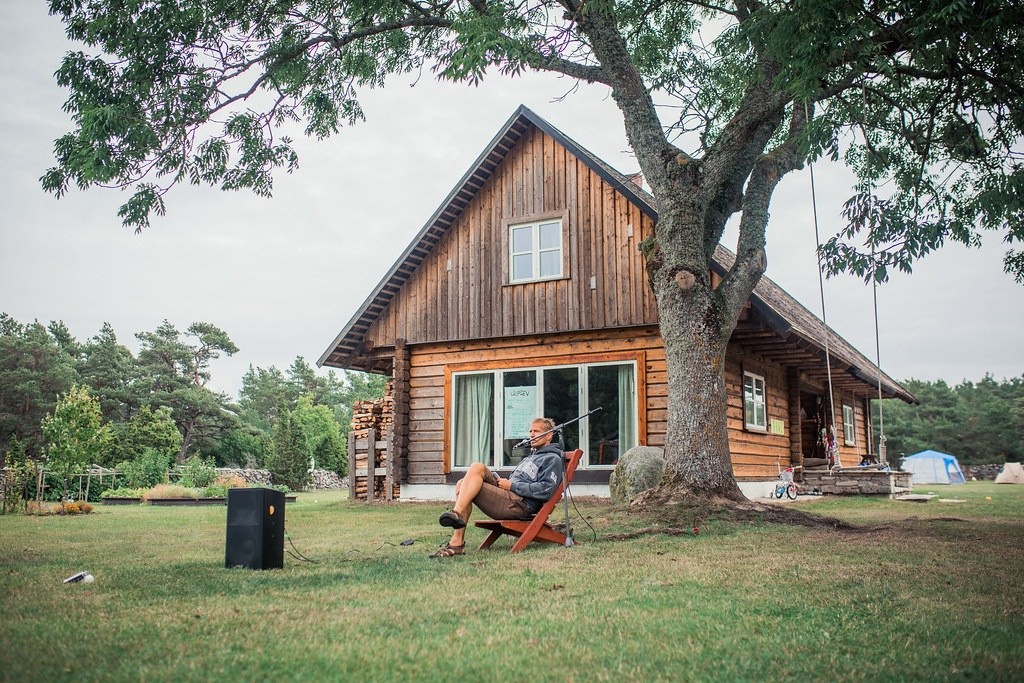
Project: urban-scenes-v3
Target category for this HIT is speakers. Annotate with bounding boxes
[225,488,285,570]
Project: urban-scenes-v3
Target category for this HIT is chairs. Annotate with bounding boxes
[599,440,618,464]
[475,447,585,553]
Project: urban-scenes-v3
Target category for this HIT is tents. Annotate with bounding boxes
[995,462,1024,484]
[899,450,967,484]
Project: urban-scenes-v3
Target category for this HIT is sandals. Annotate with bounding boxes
[429,541,466,558]
[439,510,466,529]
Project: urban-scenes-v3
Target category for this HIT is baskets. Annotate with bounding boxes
[782,472,793,482]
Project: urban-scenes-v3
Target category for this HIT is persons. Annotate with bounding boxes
[897,452,906,480]
[429,417,565,558]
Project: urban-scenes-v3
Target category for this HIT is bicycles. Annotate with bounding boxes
[769,462,802,501]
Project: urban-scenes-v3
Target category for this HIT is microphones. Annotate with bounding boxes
[513,439,528,450]
